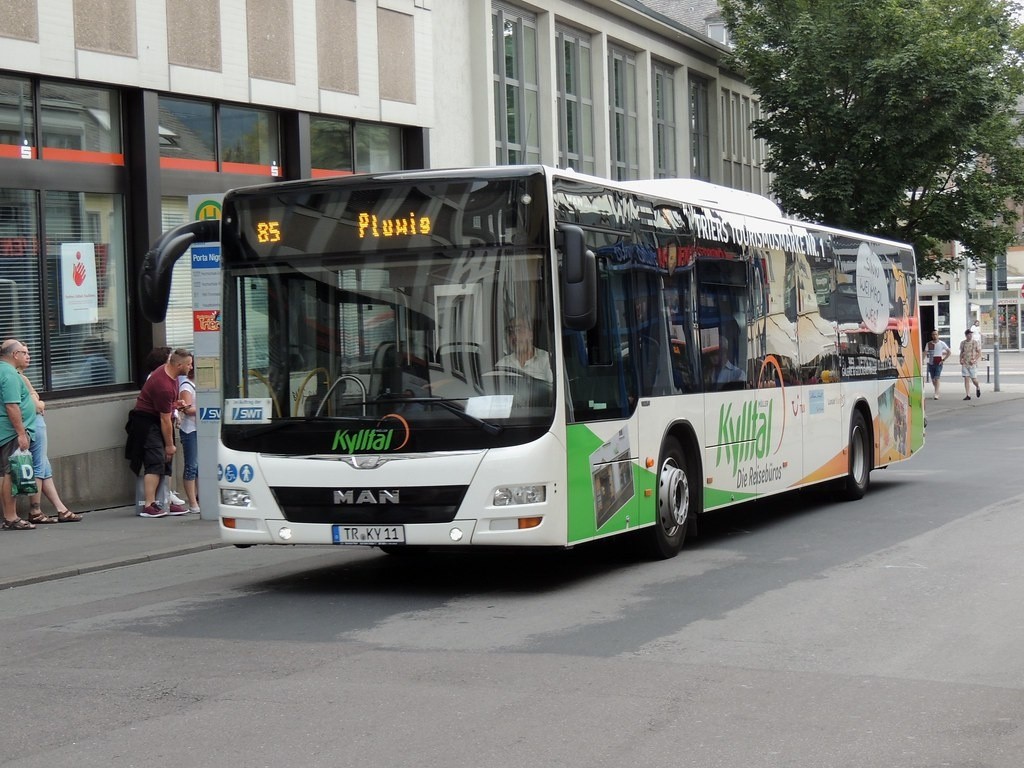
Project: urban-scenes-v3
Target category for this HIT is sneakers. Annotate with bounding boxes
[139,502,167,517]
[169,491,185,505]
[168,505,189,516]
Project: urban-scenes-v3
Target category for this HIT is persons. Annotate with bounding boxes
[921,329,951,400]
[0,339,83,531]
[970,320,983,359]
[124,347,200,517]
[700,334,745,384]
[495,315,553,405]
[959,329,981,400]
[757,367,822,388]
[66,337,114,384]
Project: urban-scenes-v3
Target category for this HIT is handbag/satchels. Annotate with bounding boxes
[8,447,38,497]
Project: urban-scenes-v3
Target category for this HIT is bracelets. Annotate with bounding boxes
[942,359,945,362]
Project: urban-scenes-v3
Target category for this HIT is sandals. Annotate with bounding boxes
[58,509,83,522]
[28,512,58,523]
[3,517,36,530]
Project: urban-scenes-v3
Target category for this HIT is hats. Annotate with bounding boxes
[965,329,973,335]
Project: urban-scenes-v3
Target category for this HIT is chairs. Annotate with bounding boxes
[436,342,484,389]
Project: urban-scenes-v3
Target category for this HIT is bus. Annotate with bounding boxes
[136,162,928,560]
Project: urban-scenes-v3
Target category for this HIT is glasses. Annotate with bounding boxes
[13,351,26,357]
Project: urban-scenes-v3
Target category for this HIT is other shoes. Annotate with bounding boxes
[963,395,971,400]
[189,507,200,514]
[934,395,938,400]
[977,388,980,398]
